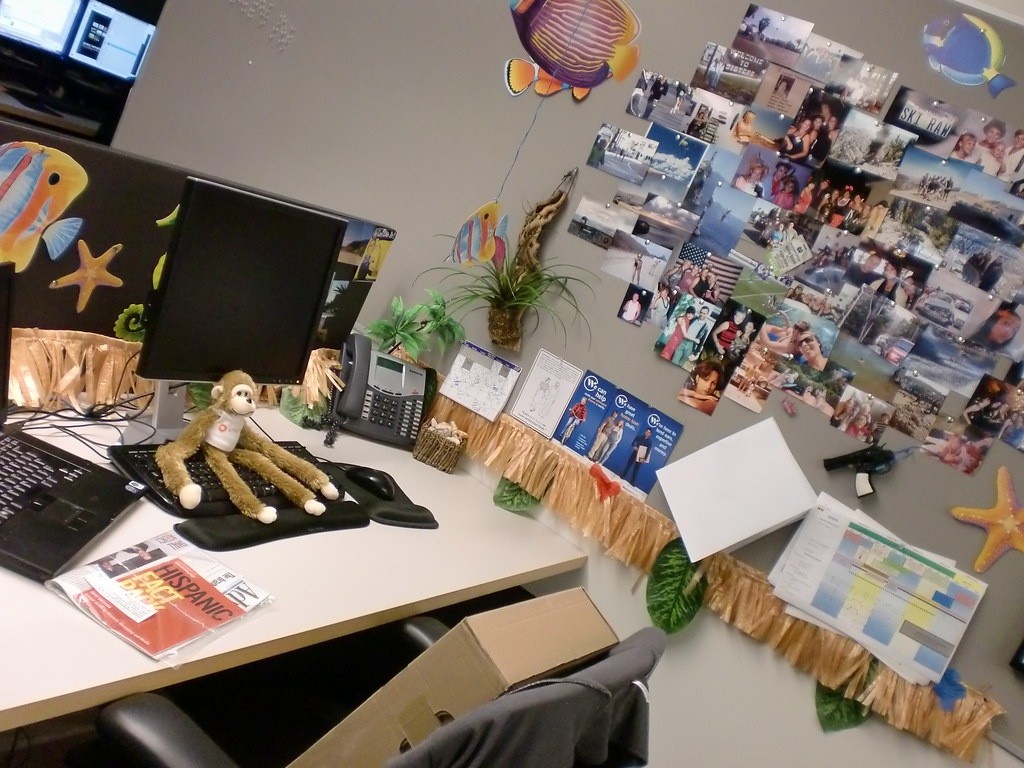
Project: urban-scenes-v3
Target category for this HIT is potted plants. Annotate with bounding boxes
[412,231,603,351]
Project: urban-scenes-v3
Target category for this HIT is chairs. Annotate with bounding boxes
[93,616,668,767]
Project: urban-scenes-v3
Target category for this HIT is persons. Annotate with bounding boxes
[559,46,1024,487]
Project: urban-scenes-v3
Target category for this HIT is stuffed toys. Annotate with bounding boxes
[157,370,340,524]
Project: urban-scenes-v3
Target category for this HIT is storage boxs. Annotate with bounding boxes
[283,586,620,767]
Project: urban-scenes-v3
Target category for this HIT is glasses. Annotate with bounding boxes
[798,336,814,345]
[792,323,805,335]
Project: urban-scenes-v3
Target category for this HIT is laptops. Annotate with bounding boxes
[0,261,151,583]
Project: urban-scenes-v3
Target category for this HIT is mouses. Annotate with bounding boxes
[345,467,396,501]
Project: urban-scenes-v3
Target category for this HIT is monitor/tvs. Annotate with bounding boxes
[0,0,348,443]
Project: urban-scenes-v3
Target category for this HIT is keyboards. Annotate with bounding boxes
[107,440,344,519]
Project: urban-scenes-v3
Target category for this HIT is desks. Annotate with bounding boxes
[0,401,586,734]
